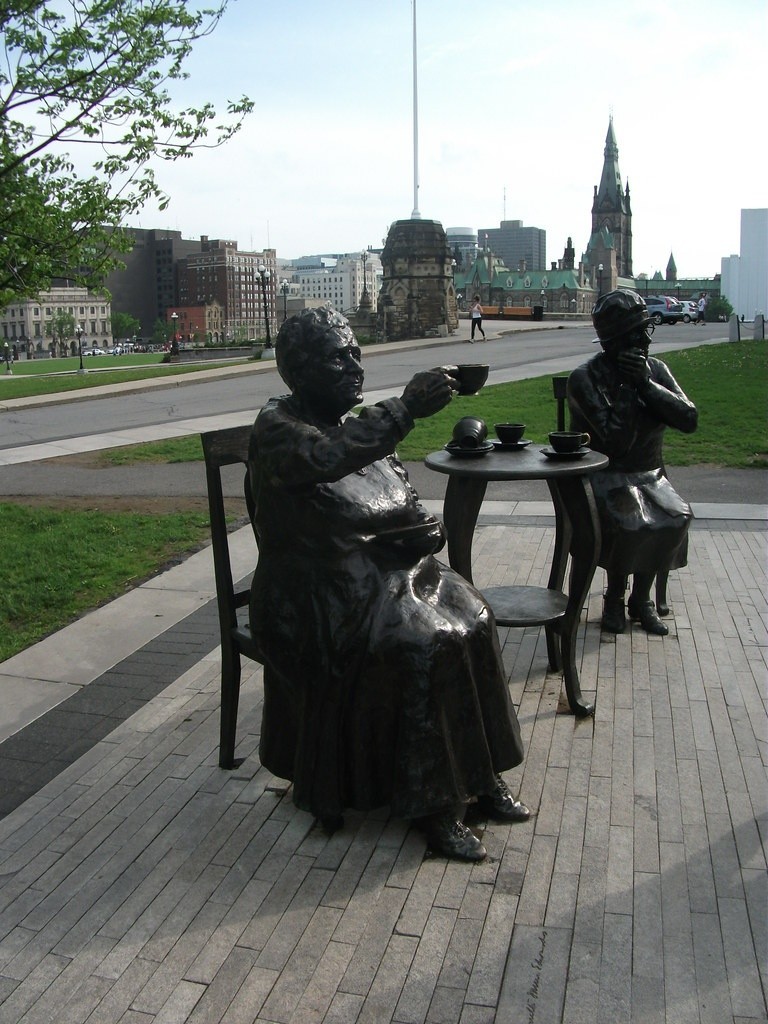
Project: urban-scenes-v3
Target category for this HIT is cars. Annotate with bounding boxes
[679,301,700,324]
[106,347,115,354]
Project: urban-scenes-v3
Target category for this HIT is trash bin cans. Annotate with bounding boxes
[533,305,543,321]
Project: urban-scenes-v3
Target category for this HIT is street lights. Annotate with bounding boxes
[450,259,456,285]
[75,324,84,369]
[457,293,463,309]
[171,312,178,339]
[281,279,290,322]
[674,282,682,300]
[254,265,274,349]
[4,342,10,370]
[360,249,371,297]
[598,264,604,295]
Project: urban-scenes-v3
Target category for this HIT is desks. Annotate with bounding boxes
[423,445,609,716]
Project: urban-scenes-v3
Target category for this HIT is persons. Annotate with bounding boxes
[693,293,707,325]
[468,296,487,344]
[562,289,697,637]
[243,308,536,863]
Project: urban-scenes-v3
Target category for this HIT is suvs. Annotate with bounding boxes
[643,296,684,325]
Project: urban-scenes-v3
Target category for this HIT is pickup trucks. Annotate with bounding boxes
[84,349,105,356]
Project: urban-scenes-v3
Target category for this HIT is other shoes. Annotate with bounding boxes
[468,339,474,344]
[483,334,486,340]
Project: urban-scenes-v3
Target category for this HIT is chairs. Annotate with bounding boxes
[199,426,271,767]
[550,380,671,630]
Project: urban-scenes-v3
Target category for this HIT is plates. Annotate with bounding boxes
[375,520,441,543]
[539,447,592,461]
[444,442,495,459]
[487,439,533,449]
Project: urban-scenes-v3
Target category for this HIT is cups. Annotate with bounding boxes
[447,363,489,397]
[447,416,489,446]
[548,431,590,452]
[494,422,525,443]
